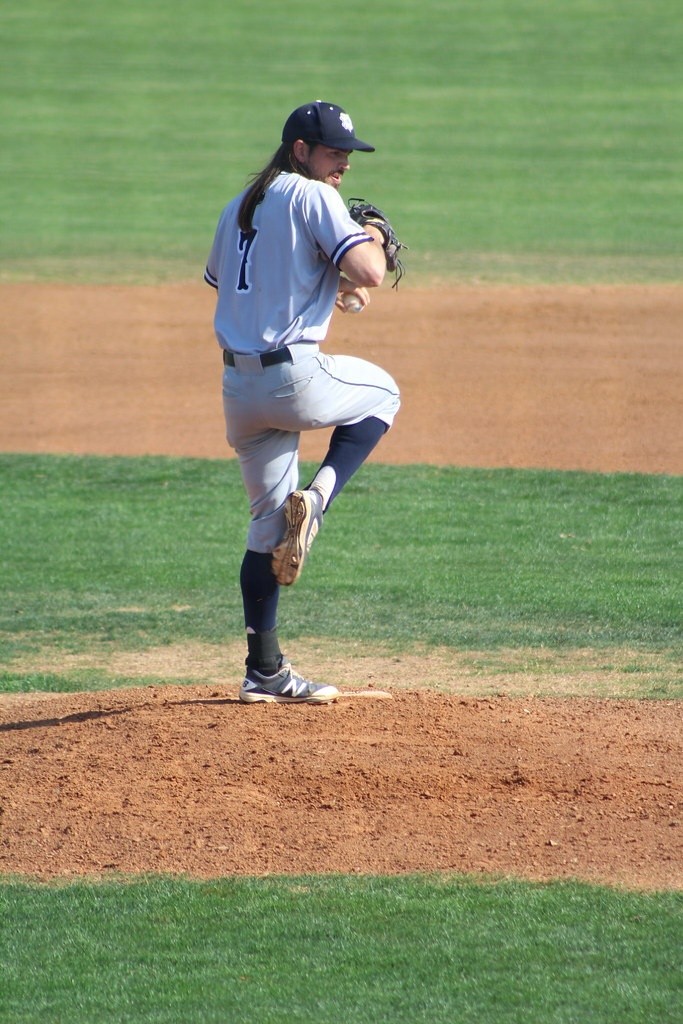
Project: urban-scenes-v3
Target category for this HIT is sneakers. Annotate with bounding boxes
[272,490,323,586]
[239,653,339,705]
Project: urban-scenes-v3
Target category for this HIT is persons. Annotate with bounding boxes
[203,100,408,702]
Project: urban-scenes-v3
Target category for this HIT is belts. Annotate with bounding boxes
[224,346,292,367]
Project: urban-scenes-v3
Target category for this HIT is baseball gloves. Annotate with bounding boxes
[349,201,400,274]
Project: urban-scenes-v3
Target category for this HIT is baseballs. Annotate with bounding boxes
[342,293,364,313]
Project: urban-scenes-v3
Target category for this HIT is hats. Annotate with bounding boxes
[282,100,375,152]
